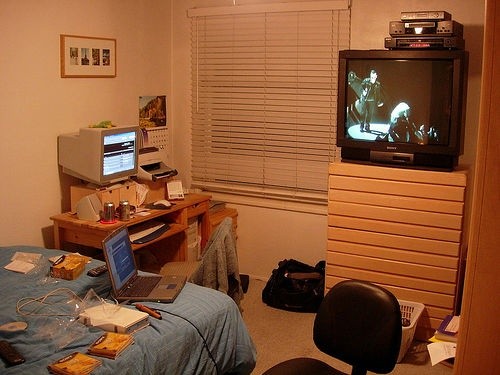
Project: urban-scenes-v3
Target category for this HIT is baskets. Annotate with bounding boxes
[396,298,425,363]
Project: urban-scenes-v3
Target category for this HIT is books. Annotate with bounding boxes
[79,303,150,333]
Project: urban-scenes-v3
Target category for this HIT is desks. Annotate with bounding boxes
[50,192,213,273]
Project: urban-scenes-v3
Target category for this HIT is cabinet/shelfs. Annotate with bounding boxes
[209,206,239,244]
[451,0,500,375]
[322,162,469,330]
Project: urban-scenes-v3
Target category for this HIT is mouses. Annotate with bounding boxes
[152,200,172,209]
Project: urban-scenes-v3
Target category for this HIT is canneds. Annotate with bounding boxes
[103,201,115,222]
[119,200,130,221]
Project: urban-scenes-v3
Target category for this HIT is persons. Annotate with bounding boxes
[391,101,418,131]
[360,70,382,131]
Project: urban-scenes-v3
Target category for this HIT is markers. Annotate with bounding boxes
[136,304,162,320]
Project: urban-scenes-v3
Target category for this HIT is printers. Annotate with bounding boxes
[134,146,179,182]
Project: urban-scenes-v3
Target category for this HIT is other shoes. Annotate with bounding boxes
[365,127,371,133]
[360,128,363,132]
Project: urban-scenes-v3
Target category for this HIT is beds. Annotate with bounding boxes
[0,245,257,375]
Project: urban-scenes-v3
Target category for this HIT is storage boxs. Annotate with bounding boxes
[119,180,139,207]
[397,298,424,366]
[70,184,120,215]
[51,254,87,281]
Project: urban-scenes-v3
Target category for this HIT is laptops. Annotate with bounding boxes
[101,224,187,303]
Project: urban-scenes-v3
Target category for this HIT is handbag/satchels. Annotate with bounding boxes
[376,134,388,141]
[263,259,325,314]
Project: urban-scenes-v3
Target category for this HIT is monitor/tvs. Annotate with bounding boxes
[335,49,469,171]
[58,123,140,190]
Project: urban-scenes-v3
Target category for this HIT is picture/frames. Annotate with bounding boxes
[59,34,118,79]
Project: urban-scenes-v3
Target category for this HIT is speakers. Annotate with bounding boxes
[119,184,137,209]
[76,191,105,222]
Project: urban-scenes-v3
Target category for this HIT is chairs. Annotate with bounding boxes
[160,216,245,319]
[259,279,403,375]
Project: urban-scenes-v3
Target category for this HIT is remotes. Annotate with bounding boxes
[88,264,108,277]
[0,340,25,365]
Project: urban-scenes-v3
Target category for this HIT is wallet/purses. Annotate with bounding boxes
[0,341,26,366]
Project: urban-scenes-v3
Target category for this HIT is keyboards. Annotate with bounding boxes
[126,219,170,244]
